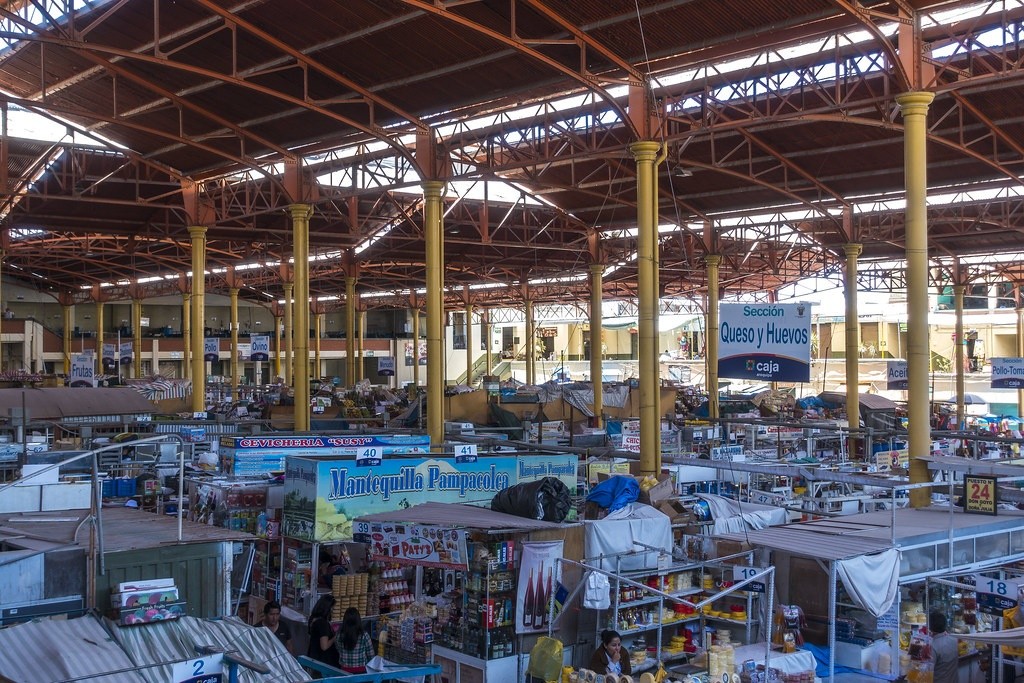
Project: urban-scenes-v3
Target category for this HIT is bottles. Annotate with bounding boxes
[440,617,512,659]
[523,560,553,629]
[359,554,416,612]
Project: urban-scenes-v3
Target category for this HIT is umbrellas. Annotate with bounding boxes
[951,394,987,429]
[972,414,1024,431]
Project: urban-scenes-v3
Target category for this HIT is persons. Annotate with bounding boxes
[590,629,632,679]
[254,395,264,405]
[584,338,590,360]
[917,613,959,683]
[445,385,451,396]
[417,388,426,401]
[207,503,216,525]
[859,447,864,457]
[335,607,374,675]
[1000,430,1022,458]
[938,414,955,440]
[980,432,998,458]
[663,350,673,358]
[306,594,341,679]
[688,350,692,359]
[252,601,292,654]
[482,339,488,349]
[694,353,699,359]
[188,504,202,521]
[307,552,346,589]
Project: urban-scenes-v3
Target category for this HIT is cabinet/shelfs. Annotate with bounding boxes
[594,569,763,674]
[251,533,519,683]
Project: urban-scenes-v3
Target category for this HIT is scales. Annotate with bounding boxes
[669,662,708,683]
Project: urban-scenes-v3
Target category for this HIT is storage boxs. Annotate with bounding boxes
[715,540,753,566]
[111,577,188,627]
[659,500,701,543]
[103,478,136,498]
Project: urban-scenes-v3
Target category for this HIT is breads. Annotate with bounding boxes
[878,600,995,675]
[561,574,816,683]
[330,573,385,622]
